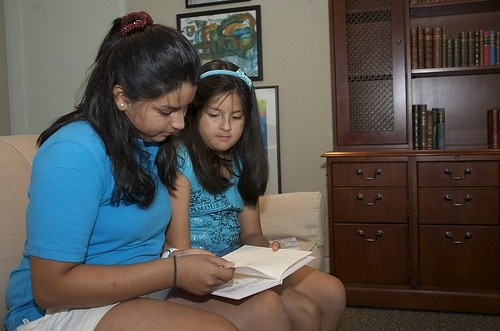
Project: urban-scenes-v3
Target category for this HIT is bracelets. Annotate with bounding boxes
[162,248,177,258]
[173,256,178,289]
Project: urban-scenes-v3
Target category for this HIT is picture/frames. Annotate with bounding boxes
[174,4,264,82]
[253,85,282,195]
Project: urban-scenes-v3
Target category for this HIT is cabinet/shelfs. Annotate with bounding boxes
[329,0,500,148]
[325,157,500,314]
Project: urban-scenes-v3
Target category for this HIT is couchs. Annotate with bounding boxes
[0,134,325,331]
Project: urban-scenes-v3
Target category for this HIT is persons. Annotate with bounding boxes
[4,11,292,331]
[164,59,348,331]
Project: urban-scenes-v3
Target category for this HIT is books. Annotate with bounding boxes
[489,109,500,148]
[410,22,500,69]
[206,242,316,300]
[412,104,445,149]
